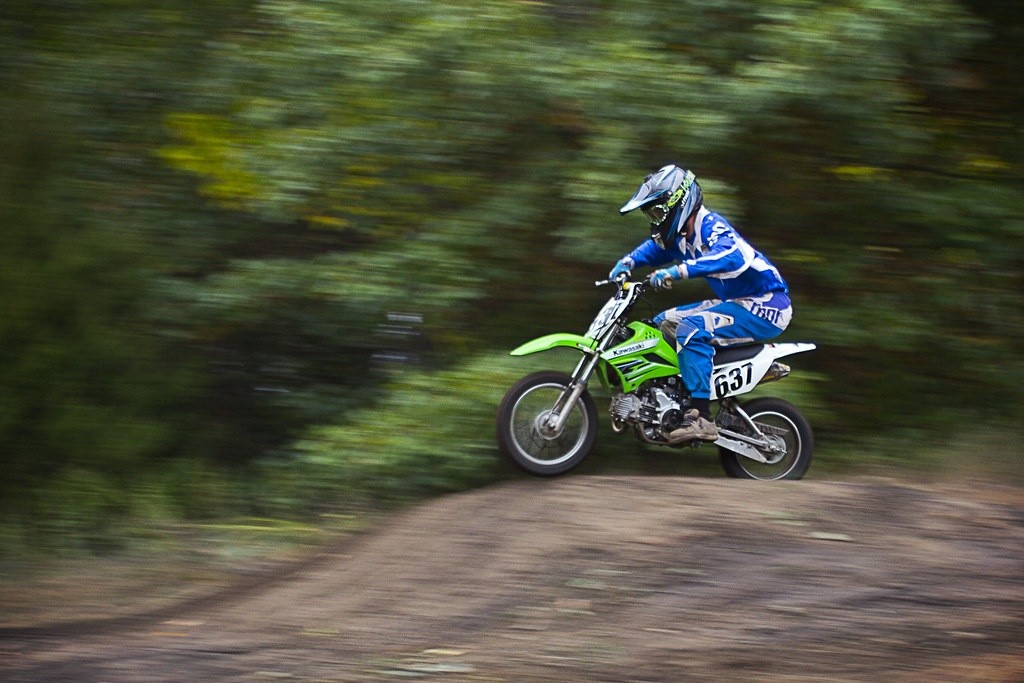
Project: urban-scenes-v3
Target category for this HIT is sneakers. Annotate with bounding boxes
[669,408,719,444]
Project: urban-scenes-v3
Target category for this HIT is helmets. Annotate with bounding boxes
[619,163,702,251]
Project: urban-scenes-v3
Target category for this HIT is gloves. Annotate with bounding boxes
[608,257,635,284]
[647,264,682,294]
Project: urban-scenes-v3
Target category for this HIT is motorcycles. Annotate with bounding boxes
[491,275,815,485]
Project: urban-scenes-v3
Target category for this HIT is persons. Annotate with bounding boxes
[610,165,792,441]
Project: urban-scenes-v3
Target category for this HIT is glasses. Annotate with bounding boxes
[641,205,669,226]
